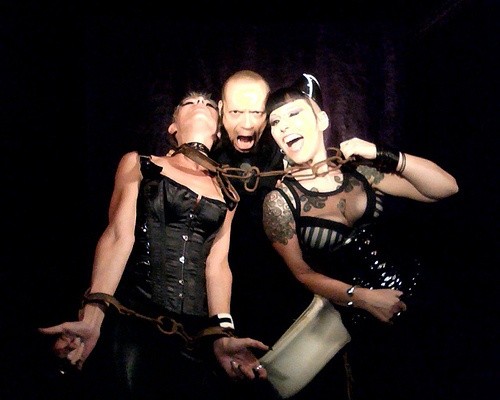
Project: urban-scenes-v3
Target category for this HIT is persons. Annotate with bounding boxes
[36,71,459,383]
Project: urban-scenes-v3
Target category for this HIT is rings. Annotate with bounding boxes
[255,365,262,371]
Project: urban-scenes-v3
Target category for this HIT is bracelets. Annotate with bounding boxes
[78,293,116,320]
[398,152,406,174]
[200,327,233,338]
[204,313,235,329]
[370,142,399,171]
[347,284,360,307]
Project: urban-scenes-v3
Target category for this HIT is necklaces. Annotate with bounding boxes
[172,141,209,157]
